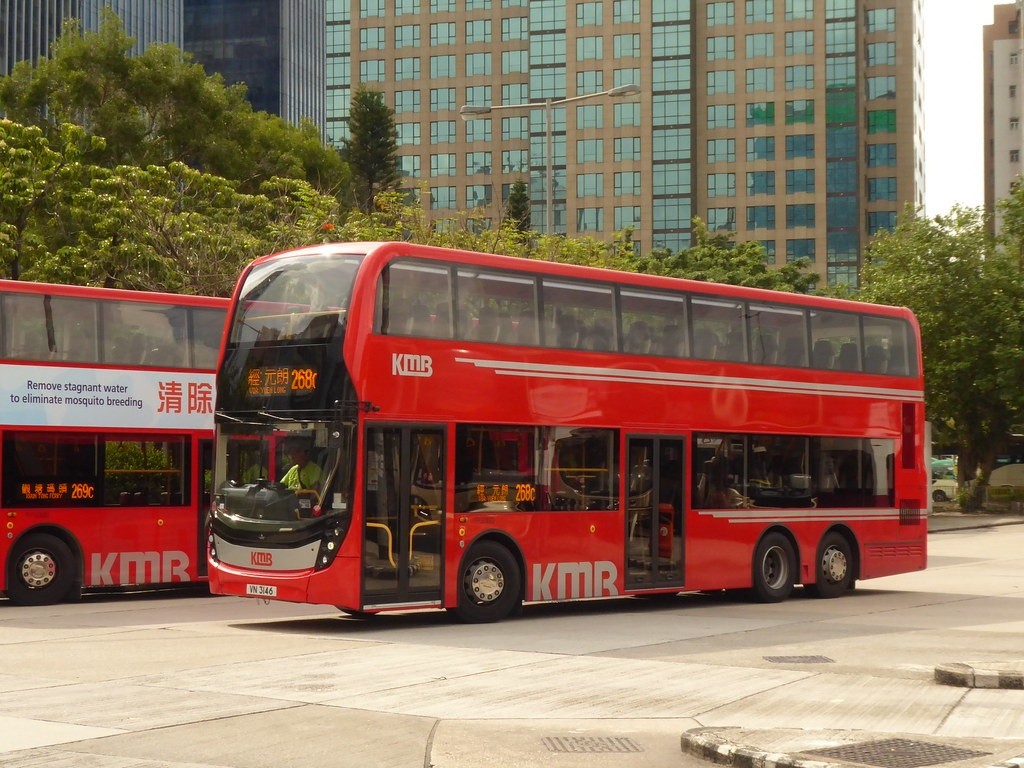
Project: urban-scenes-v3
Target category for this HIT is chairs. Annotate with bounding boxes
[383,296,912,377]
[21,330,184,369]
[121,490,172,507]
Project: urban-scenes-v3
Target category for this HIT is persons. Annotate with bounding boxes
[280,445,324,516]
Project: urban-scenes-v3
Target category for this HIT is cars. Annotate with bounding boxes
[930,455,958,502]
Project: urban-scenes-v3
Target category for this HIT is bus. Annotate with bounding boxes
[0,277,527,606]
[204,239,929,626]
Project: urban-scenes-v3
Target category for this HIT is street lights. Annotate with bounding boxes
[458,83,644,259]
